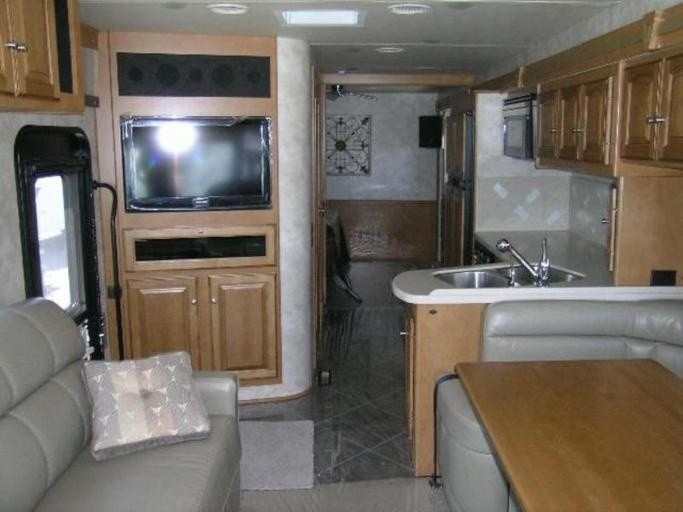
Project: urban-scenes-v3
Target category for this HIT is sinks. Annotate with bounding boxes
[436,272,511,289]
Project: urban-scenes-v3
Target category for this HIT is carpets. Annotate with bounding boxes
[237,419,317,493]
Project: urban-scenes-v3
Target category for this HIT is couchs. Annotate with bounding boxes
[434,300,682,512]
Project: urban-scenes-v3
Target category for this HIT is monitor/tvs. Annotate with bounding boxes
[120,114,273,212]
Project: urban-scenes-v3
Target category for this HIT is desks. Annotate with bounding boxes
[453,357,682,512]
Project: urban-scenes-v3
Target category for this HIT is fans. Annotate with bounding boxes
[324,85,378,102]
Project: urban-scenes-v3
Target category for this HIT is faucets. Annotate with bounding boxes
[496,238,550,287]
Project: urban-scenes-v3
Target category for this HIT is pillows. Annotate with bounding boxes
[81,350,215,462]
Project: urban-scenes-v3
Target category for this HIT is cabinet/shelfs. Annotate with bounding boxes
[0,0,61,112]
[400,301,488,478]
[619,3,683,170]
[537,28,623,178]
[119,266,282,388]
[118,223,277,274]
[600,176,682,286]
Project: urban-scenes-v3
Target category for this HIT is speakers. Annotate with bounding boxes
[418,115,442,149]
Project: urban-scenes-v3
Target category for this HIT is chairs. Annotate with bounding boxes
[0,296,244,512]
